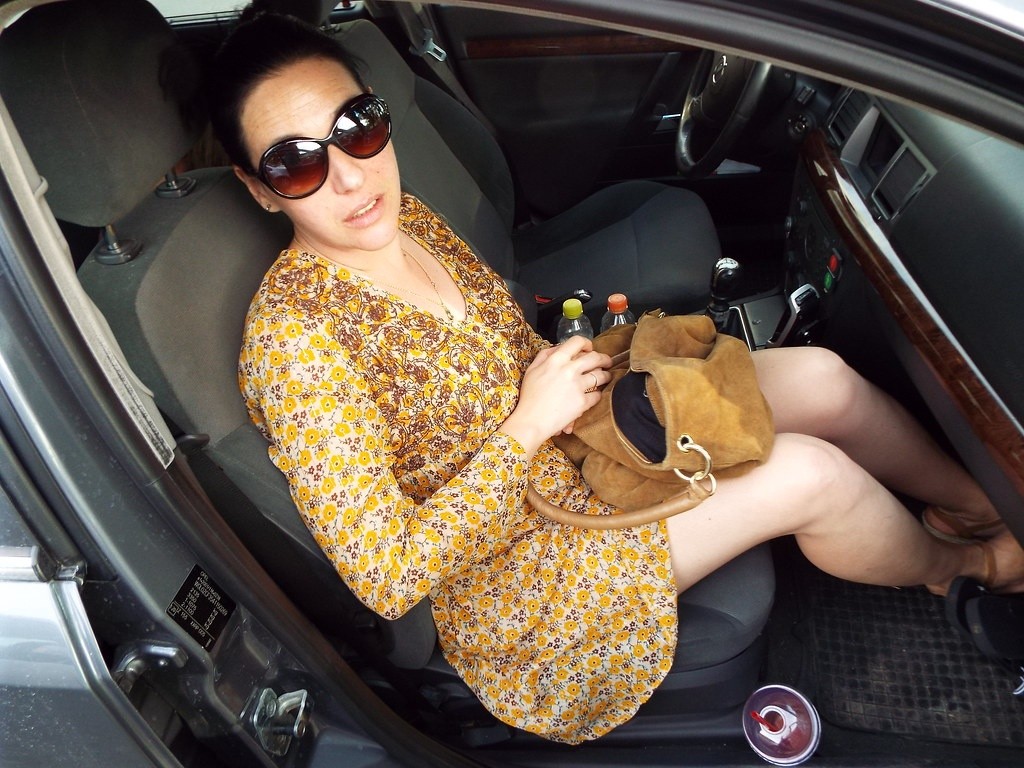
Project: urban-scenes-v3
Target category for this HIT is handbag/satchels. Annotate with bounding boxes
[521,312,775,525]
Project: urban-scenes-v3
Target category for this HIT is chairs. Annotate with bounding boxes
[250,0,720,339]
[2,0,776,677]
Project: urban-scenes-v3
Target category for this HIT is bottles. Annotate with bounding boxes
[601,293,636,334]
[557,298,594,344]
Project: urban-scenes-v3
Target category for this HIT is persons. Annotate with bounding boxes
[157,10,1024,746]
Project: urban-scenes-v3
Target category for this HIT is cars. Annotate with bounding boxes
[0,1,1024,767]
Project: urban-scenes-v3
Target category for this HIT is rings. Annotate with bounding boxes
[585,372,597,392]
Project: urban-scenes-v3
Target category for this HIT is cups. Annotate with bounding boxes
[741,684,822,767]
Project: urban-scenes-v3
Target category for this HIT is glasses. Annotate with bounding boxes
[248,92,397,201]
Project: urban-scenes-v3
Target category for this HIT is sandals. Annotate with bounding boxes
[923,538,1024,597]
[923,503,1007,542]
